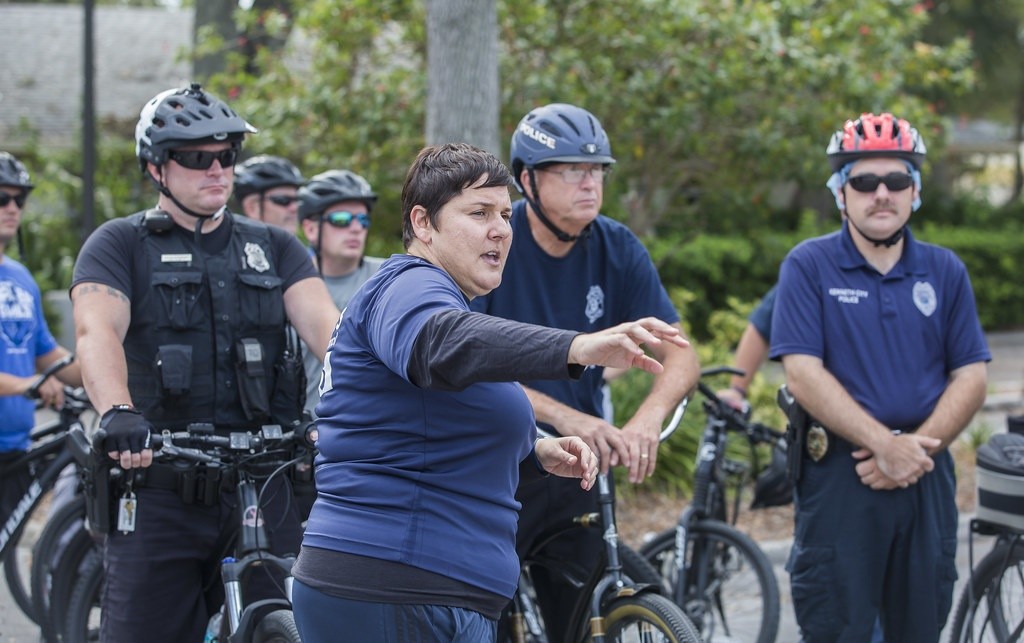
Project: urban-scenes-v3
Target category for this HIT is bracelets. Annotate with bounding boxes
[729,387,746,398]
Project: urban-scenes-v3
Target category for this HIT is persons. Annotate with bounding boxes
[0,152,82,527]
[473,104,699,643]
[295,170,390,419]
[714,284,784,409]
[72,84,342,643]
[769,111,992,643]
[292,145,691,643]
[233,153,318,272]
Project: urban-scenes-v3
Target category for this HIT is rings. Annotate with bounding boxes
[640,454,649,458]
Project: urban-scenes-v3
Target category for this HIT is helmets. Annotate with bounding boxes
[0,151,34,192]
[134,83,259,173]
[510,103,617,183]
[826,112,927,173]
[234,156,307,207]
[297,169,379,225]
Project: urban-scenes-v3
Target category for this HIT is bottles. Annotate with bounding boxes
[202,603,227,643]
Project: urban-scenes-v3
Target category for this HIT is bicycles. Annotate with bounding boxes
[0,355,317,643]
[636,366,795,643]
[498,395,701,643]
[947,415,1024,643]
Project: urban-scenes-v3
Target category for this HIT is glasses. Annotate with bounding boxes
[269,195,297,206]
[848,171,914,193]
[168,148,235,170]
[323,210,372,227]
[0,192,25,208]
[544,164,613,183]
